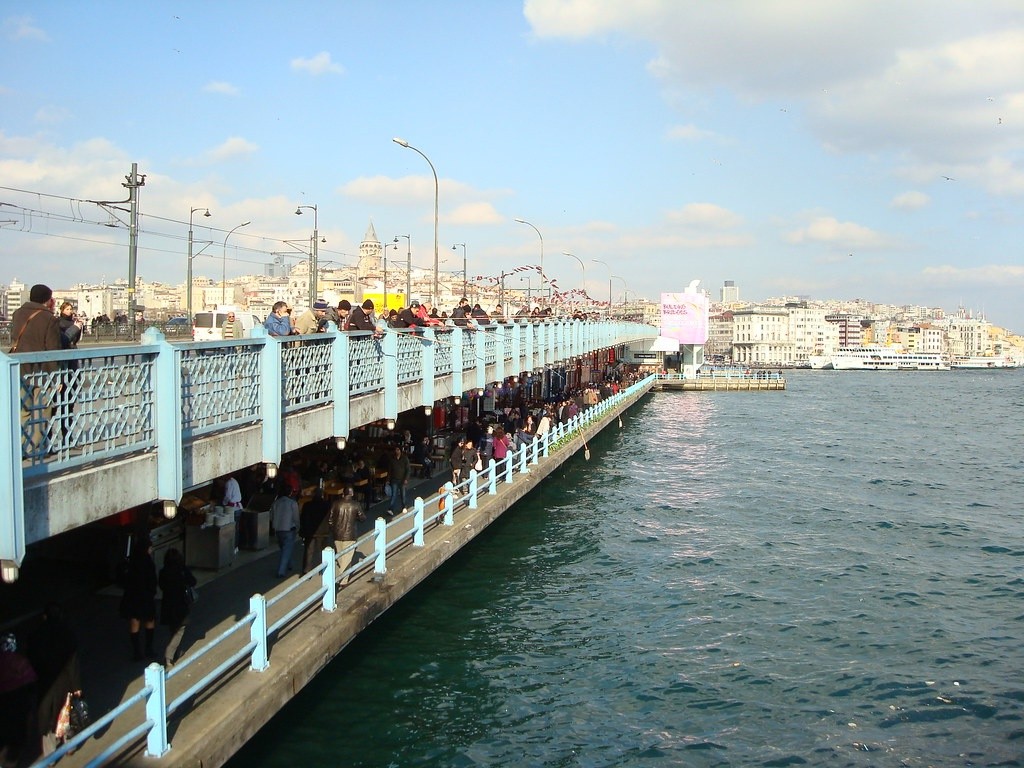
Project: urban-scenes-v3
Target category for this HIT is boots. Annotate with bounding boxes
[131,628,154,662]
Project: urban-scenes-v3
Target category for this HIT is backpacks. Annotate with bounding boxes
[481,441,493,458]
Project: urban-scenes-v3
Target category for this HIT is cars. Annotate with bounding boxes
[164,317,188,333]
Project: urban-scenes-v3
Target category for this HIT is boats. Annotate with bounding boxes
[808,343,1017,371]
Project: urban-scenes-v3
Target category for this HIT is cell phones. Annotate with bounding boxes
[277,310,282,316]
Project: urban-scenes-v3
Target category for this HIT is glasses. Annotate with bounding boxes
[228,316,235,318]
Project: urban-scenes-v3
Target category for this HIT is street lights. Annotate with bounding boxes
[384,242,398,317]
[452,243,467,299]
[514,217,544,310]
[593,259,612,319]
[610,275,637,315]
[561,252,586,290]
[393,234,411,307]
[188,206,212,330]
[308,235,327,311]
[392,137,438,308]
[520,276,530,311]
[294,204,318,304]
[222,221,251,304]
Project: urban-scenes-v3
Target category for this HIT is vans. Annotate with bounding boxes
[191,312,262,357]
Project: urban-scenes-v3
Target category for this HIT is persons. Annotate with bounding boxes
[158,548,198,667]
[222,312,243,376]
[743,369,783,379]
[298,487,333,579]
[119,546,157,661]
[663,369,667,379]
[710,368,714,379]
[264,297,658,515]
[269,484,300,580]
[0,601,77,768]
[206,474,243,555]
[328,487,367,586]
[10,285,85,457]
[696,368,700,379]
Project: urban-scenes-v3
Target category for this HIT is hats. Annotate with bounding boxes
[313,299,328,311]
[362,299,374,310]
[339,300,351,311]
[389,309,398,318]
[30,285,52,303]
[410,301,421,308]
[463,305,471,312]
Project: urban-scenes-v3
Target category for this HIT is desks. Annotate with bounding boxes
[300,471,388,503]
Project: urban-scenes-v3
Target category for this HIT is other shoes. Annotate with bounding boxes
[162,656,173,667]
[63,441,83,450]
[402,508,407,513]
[387,510,393,516]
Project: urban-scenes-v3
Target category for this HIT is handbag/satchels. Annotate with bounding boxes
[385,483,393,497]
[55,691,90,740]
[9,346,16,353]
[184,587,198,605]
[475,453,482,471]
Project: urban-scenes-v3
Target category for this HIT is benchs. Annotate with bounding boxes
[409,461,423,477]
[431,456,444,471]
